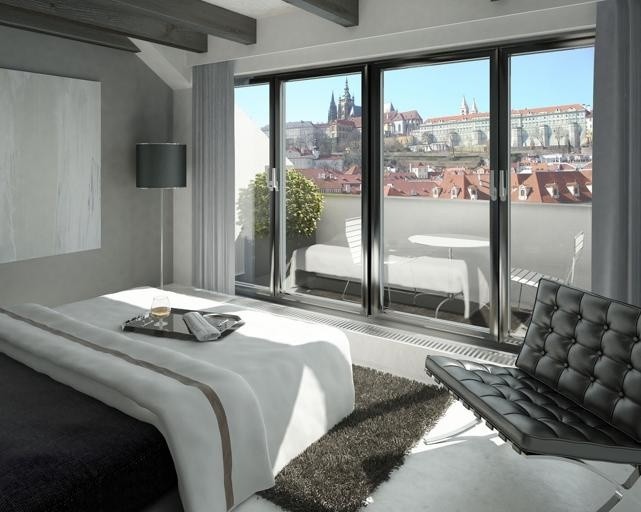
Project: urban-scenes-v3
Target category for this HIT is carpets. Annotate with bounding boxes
[254,361,457,512]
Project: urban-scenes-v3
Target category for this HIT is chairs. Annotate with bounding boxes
[508,230,587,316]
[340,217,396,306]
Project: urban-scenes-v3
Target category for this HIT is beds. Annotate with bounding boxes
[1,283,357,512]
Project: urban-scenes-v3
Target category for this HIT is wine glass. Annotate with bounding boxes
[151,296,171,327]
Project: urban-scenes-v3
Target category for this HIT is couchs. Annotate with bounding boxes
[424,275,641,511]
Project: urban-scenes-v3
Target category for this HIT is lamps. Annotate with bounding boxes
[135,141,188,282]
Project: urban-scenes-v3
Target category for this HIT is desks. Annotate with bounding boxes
[409,233,491,318]
